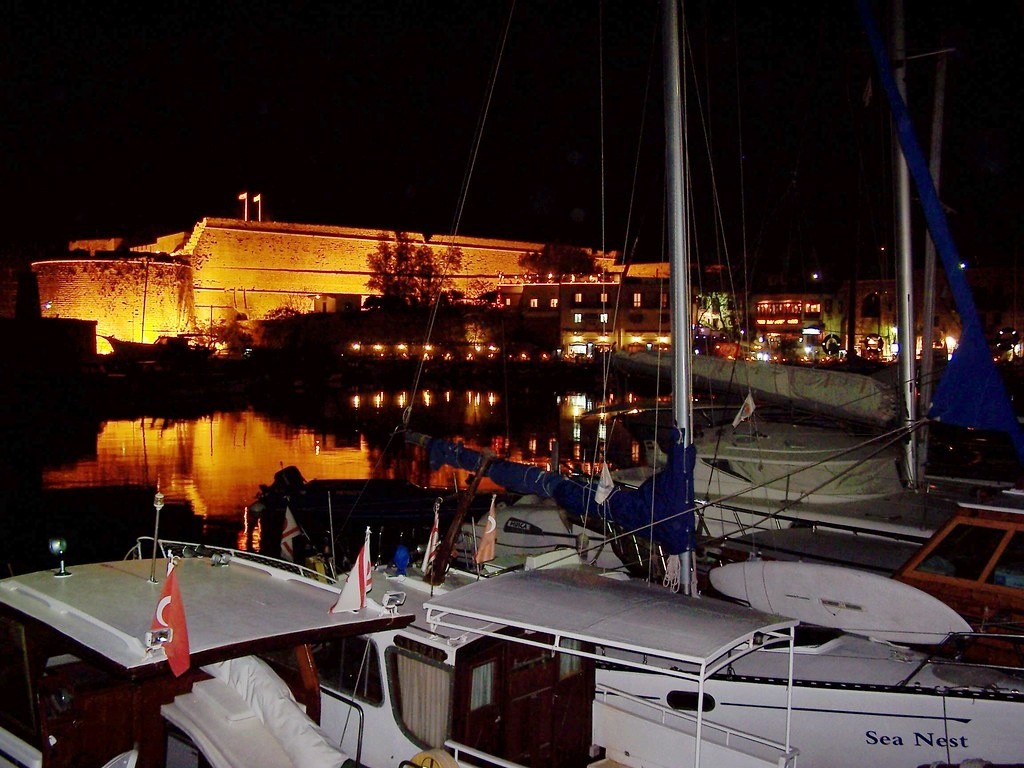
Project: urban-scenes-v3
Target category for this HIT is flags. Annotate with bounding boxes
[595,461,614,505]
[420,514,442,573]
[149,562,190,678]
[278,508,301,559]
[328,533,376,614]
[473,502,495,563]
[733,394,755,428]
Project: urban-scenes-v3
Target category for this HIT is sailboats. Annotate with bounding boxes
[2,1,1024,765]
